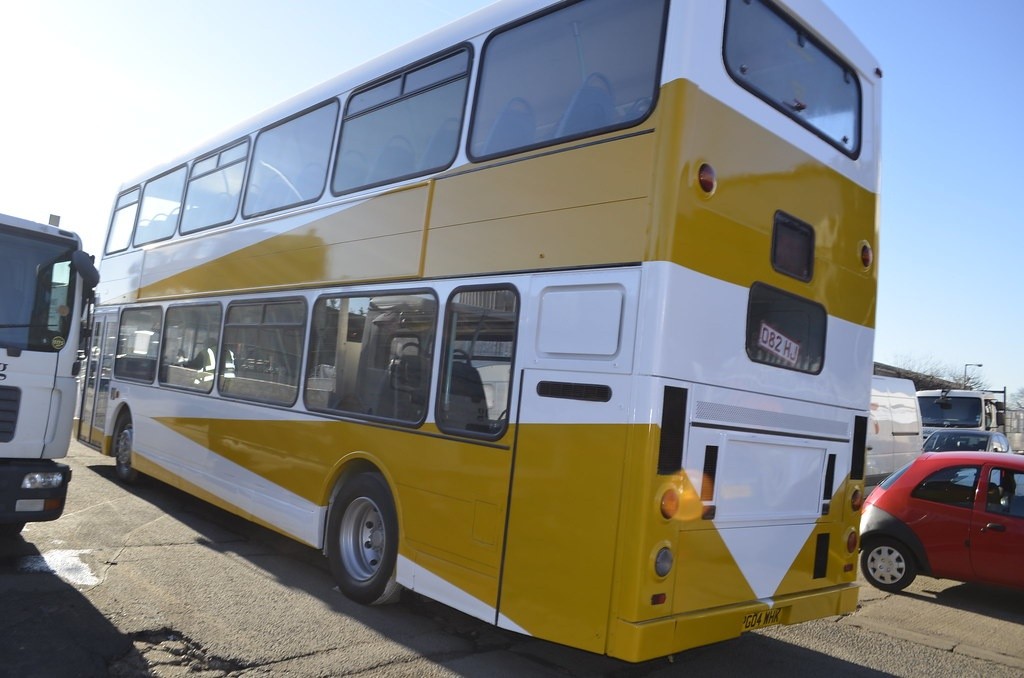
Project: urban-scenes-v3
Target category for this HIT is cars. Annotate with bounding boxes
[922,429,1015,458]
[856,450,1024,602]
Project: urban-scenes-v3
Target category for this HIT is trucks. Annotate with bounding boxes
[859,374,922,510]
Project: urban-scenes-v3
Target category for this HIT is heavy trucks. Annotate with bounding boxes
[0,209,101,537]
[914,386,998,447]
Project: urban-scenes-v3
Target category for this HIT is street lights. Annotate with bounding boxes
[963,363,984,390]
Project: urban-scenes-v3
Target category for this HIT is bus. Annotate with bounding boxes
[79,0,883,662]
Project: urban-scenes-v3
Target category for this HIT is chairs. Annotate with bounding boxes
[129,70,653,250]
[914,478,1024,518]
[371,338,489,434]
